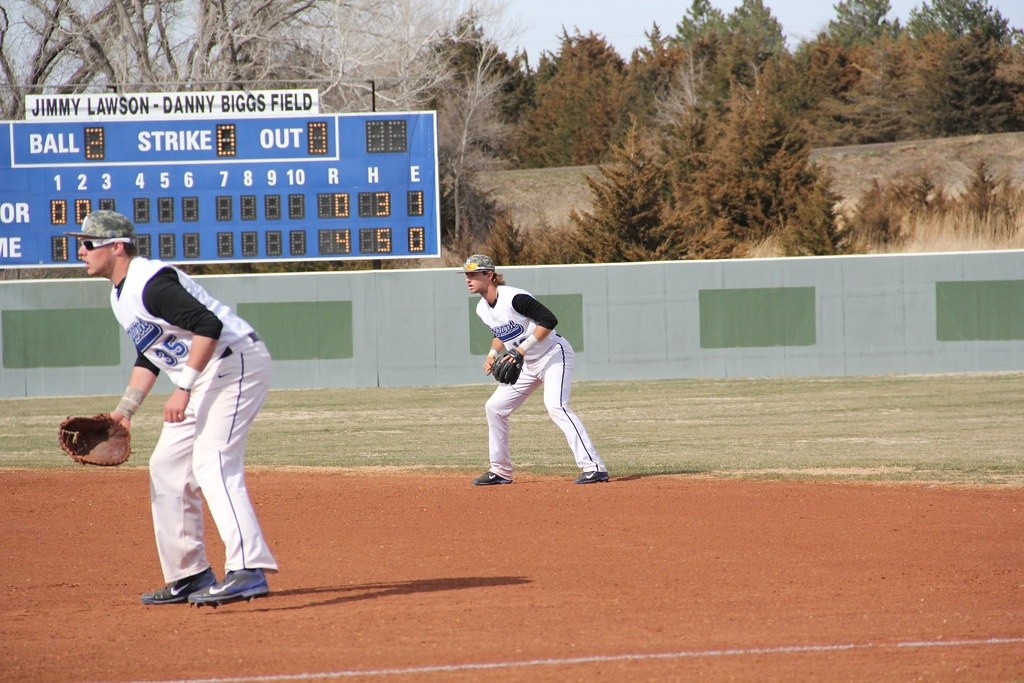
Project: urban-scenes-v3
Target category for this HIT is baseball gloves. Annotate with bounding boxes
[489,349,525,385]
[58,411,134,466]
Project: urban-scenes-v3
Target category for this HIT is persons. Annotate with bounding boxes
[62,210,281,606]
[456,253,609,485]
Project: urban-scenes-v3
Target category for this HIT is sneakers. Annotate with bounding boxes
[189,567,269,605]
[472,472,513,485]
[141,566,217,604]
[574,471,608,484]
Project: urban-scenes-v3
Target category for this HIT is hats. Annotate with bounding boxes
[459,253,495,274]
[65,210,136,244]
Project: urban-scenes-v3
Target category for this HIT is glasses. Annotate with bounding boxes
[80,238,130,251]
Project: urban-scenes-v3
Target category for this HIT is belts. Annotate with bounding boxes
[220,331,261,359]
